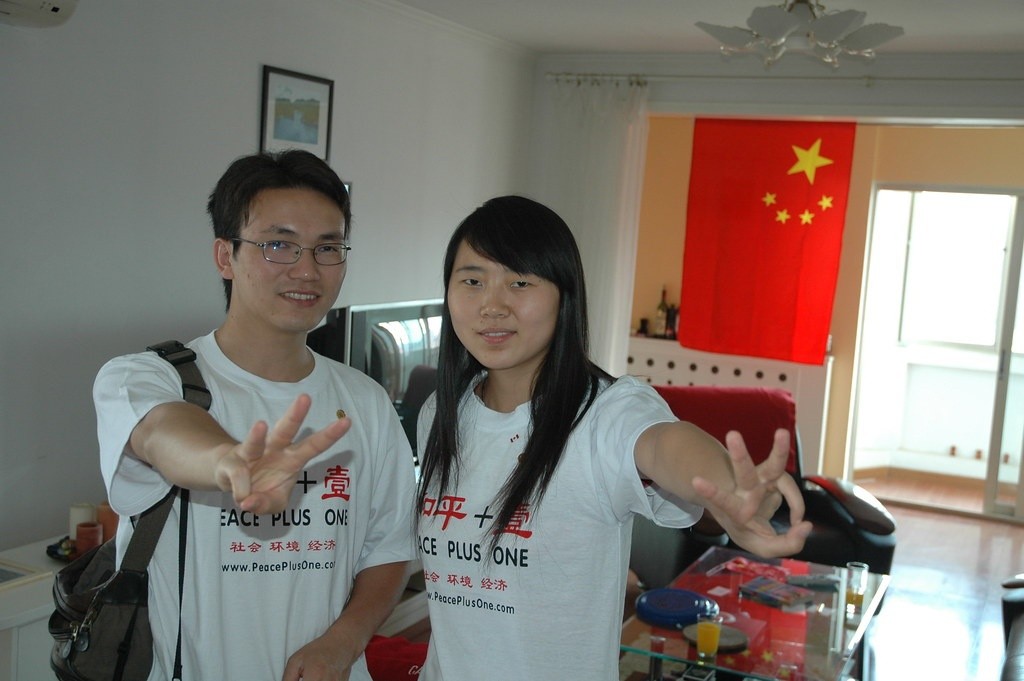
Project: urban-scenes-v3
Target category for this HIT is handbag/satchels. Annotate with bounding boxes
[48,339,211,681]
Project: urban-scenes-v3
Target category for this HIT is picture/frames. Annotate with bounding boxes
[258,65,334,162]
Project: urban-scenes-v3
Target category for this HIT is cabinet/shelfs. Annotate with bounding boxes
[0,533,69,681]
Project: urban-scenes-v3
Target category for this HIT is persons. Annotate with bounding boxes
[93,144,424,681]
[409,194,813,681]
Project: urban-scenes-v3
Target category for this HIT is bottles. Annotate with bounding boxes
[653,285,667,337]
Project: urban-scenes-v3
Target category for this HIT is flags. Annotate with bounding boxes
[676,116,858,366]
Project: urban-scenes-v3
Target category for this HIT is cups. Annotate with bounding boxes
[97,503,119,544]
[845,562,869,602]
[69,502,94,540]
[75,522,103,556]
[696,613,723,660]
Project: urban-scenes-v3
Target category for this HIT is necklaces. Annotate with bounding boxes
[482,375,488,403]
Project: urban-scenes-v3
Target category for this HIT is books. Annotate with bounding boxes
[739,576,815,606]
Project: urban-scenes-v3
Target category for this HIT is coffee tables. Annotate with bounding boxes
[620,546,893,681]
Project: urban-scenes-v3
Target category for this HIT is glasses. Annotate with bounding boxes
[231,236,352,266]
